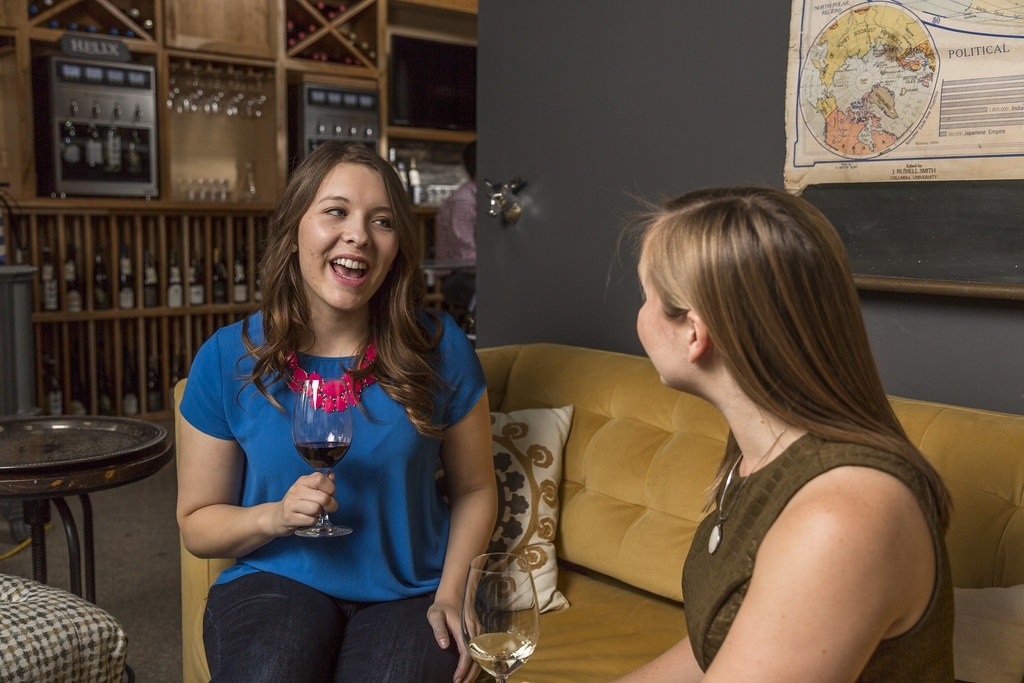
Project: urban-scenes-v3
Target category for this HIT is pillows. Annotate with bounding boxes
[953,583,1024,683]
[437,404,570,613]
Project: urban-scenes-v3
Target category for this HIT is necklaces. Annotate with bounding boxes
[281,324,382,414]
[707,421,790,554]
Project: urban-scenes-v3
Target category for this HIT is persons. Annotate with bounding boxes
[613,186,957,683]
[176,141,499,683]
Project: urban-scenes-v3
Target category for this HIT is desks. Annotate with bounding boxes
[1,415,173,606]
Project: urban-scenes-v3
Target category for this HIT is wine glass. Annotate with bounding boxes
[461,552,541,683]
[294,379,354,538]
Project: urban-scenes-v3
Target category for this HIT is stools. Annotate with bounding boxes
[1,572,128,683]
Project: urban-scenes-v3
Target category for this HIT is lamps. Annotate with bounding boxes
[481,175,526,225]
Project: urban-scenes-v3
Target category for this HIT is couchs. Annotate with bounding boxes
[173,341,1024,682]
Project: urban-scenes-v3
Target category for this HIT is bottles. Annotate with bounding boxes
[16,244,262,311]
[46,351,181,417]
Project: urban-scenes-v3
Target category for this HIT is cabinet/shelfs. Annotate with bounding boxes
[0,0,479,428]
